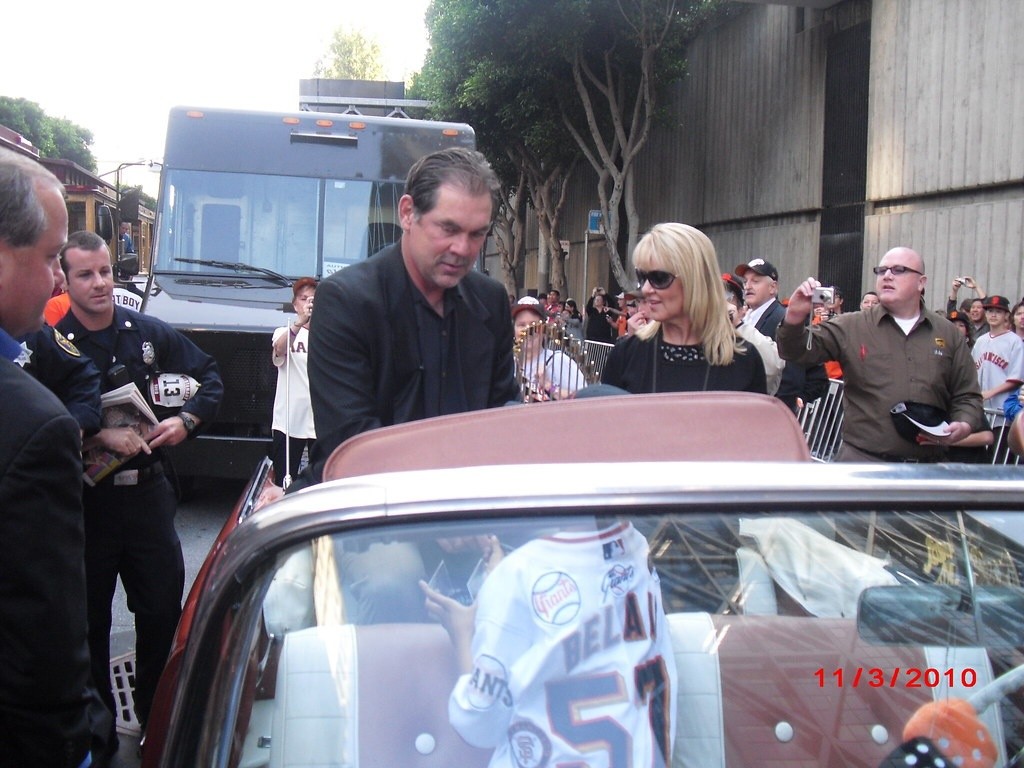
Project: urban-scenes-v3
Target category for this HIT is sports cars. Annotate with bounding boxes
[140,390,1024,768]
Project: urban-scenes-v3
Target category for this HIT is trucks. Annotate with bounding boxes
[112,105,476,501]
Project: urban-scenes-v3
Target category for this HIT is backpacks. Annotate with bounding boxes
[801,362,829,403]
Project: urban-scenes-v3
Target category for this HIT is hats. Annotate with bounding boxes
[948,311,970,324]
[723,272,744,290]
[627,301,636,307]
[512,296,545,318]
[615,292,624,299]
[293,277,318,294]
[982,296,1011,312]
[831,285,843,294]
[890,401,951,440]
[961,299,973,310]
[735,258,778,281]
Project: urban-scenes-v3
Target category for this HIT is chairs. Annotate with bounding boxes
[269,611,517,767]
[663,610,951,768]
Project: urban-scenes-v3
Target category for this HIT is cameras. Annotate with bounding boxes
[958,279,967,285]
[812,286,836,304]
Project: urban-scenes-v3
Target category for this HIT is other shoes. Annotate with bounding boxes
[88,751,112,768]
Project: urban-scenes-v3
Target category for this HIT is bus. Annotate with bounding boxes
[0,124,156,313]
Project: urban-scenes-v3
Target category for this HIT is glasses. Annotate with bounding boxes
[635,268,676,290]
[873,265,922,275]
[835,295,842,298]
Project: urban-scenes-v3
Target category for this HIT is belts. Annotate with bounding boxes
[104,460,163,487]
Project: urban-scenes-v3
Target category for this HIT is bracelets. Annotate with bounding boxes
[294,321,298,326]
[290,329,296,336]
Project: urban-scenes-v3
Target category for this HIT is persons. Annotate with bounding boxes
[272,277,319,487]
[540,288,652,342]
[777,246,981,573]
[723,259,1024,466]
[0,147,225,768]
[511,296,587,404]
[599,222,767,613]
[419,385,675,768]
[286,148,521,629]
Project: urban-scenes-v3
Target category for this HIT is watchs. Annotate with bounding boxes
[178,413,194,435]
